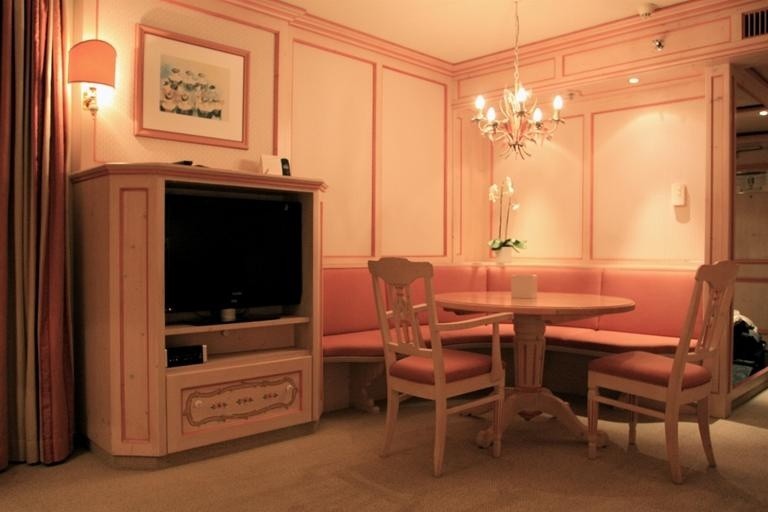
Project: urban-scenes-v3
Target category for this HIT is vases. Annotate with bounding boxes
[491,244,516,267]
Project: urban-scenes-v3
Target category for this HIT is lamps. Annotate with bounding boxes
[469,0,569,160]
[62,36,121,119]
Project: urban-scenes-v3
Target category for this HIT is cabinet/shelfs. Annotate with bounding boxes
[69,160,327,469]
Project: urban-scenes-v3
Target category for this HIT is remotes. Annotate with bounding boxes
[281,158,290,176]
[173,161,192,165]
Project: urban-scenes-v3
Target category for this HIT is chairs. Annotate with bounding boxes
[584,256,745,485]
[365,254,516,479]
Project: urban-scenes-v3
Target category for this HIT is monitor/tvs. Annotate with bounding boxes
[165,192,302,326]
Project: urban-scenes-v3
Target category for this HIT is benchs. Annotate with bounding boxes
[320,255,711,417]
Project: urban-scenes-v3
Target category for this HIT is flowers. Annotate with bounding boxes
[485,173,524,255]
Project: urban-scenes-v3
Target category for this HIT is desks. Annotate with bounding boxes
[430,290,637,454]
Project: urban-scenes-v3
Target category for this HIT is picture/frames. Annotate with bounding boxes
[128,20,252,153]
